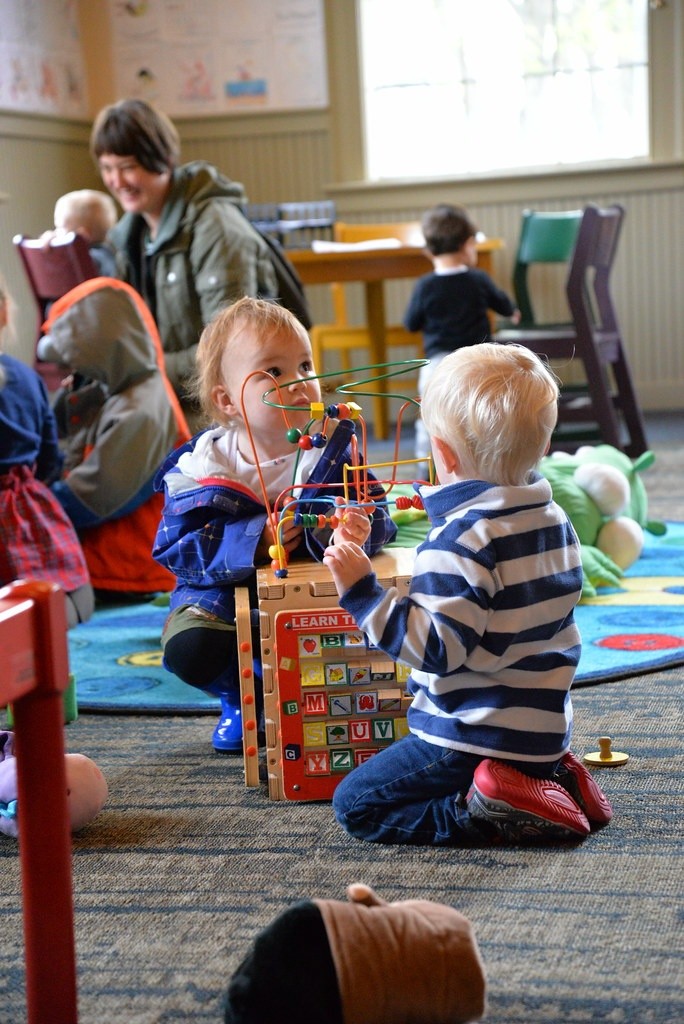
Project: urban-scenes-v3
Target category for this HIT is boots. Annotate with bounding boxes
[162,655,262,750]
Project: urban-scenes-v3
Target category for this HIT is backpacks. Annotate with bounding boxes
[186,196,312,330]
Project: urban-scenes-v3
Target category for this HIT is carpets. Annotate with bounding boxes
[62,515,684,719]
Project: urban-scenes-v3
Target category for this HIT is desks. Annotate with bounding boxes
[280,236,505,444]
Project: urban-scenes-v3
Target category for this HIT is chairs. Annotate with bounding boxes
[491,199,651,457]
[485,204,607,432]
[12,229,102,395]
[309,219,426,406]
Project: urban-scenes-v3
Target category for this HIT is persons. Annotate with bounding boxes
[39,189,117,279]
[39,277,192,608]
[91,98,280,439]
[0,280,94,626]
[405,201,521,493]
[151,297,398,750]
[321,343,615,846]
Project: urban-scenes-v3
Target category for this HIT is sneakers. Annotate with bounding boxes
[534,750,614,824]
[456,758,591,842]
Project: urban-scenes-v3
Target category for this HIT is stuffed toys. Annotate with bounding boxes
[539,443,667,596]
[0,730,109,839]
[224,881,486,1024]
